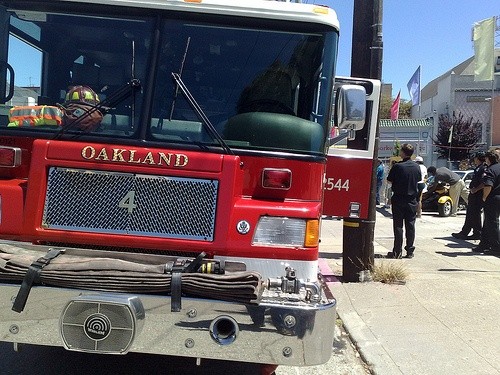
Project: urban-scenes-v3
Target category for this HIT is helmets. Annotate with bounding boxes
[65,85,100,104]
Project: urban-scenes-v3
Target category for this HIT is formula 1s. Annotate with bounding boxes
[422,180,469,216]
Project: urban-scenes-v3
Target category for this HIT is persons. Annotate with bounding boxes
[386,143,422,259]
[245,305,316,340]
[427,166,471,217]
[376,156,427,217]
[452,147,500,255]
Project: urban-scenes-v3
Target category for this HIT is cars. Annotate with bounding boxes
[452,170,474,186]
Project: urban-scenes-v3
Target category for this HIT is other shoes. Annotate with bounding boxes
[450,213,456,217]
[387,252,402,259]
[452,231,467,239]
[406,251,414,258]
[484,248,500,255]
[382,205,390,209]
[472,245,488,252]
[466,234,482,240]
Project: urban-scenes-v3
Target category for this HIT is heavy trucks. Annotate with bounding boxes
[0,0,381,368]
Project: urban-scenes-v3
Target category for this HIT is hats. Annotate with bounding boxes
[413,155,423,161]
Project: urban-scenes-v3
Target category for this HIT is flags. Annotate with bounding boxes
[390,92,401,121]
[473,15,495,81]
[407,66,422,107]
[330,125,335,138]
